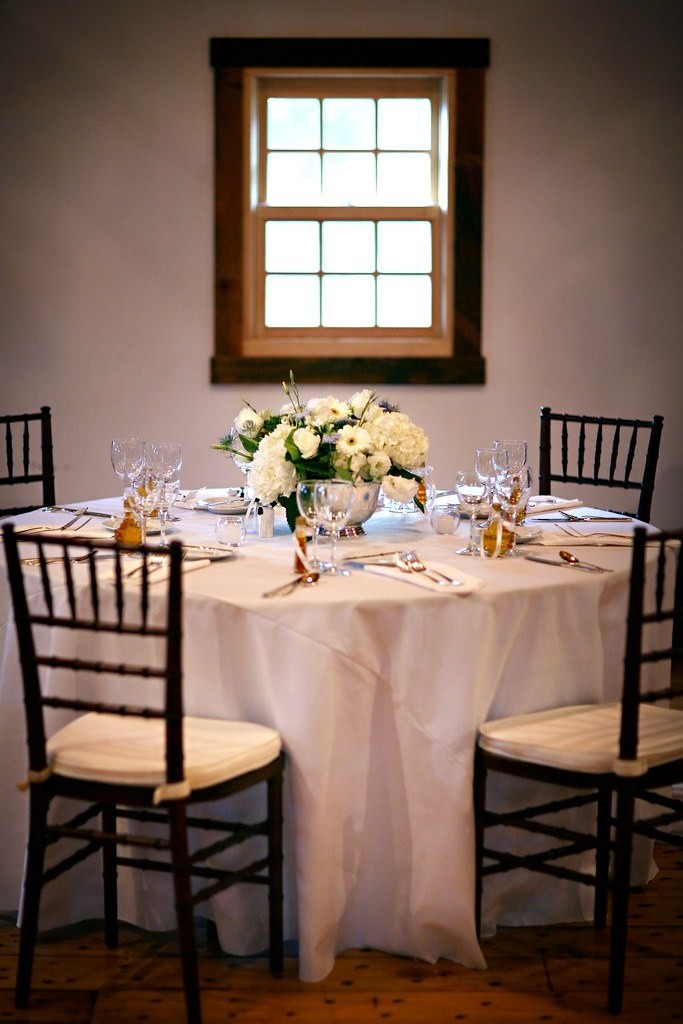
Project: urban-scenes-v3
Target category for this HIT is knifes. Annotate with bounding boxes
[525,556,602,572]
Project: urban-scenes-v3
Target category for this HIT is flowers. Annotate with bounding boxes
[213,369,429,541]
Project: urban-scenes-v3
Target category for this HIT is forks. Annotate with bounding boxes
[17,507,93,533]
[559,551,613,572]
[559,510,632,521]
[395,553,461,585]
[181,487,199,503]
[30,550,98,566]
[120,544,168,578]
[555,524,632,540]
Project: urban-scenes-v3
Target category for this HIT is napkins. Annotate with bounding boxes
[5,520,111,540]
[100,556,211,586]
[170,492,210,512]
[365,557,483,592]
[524,532,682,546]
[525,497,583,514]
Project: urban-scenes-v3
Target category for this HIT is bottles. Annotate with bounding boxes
[136,468,158,519]
[483,503,514,558]
[296,518,306,573]
[506,477,526,525]
[114,498,141,547]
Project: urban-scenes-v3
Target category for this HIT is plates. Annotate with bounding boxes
[102,519,175,535]
[185,545,235,561]
[198,496,254,514]
[515,526,542,543]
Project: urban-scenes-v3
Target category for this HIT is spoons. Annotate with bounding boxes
[264,573,319,597]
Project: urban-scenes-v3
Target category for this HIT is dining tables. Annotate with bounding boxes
[1,488,675,983]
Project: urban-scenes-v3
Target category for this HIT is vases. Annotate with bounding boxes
[316,479,380,538]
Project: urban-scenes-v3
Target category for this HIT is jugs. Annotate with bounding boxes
[387,467,436,513]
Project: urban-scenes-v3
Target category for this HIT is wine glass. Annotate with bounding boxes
[111,438,183,558]
[315,480,353,575]
[455,440,531,556]
[297,481,333,573]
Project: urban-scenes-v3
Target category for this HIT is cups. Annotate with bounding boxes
[431,504,460,535]
[216,516,246,547]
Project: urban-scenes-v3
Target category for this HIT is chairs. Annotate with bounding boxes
[473,524,683,1013]
[0,406,56,517]
[539,406,665,524]
[2,523,286,1024]
